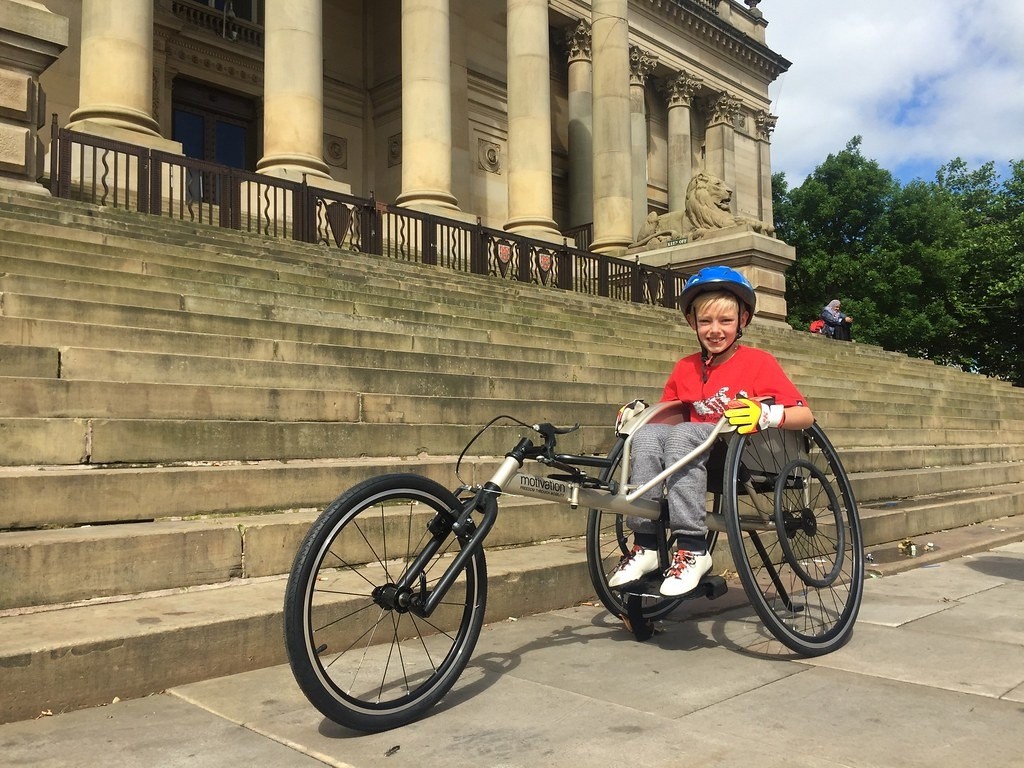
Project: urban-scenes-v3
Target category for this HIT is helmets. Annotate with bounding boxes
[678,266,757,327]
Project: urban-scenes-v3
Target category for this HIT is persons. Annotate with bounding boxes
[609,265,814,600]
[816,299,854,342]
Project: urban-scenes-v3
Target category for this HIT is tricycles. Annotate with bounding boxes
[283,387,865,734]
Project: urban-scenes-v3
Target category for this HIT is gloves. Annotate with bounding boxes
[615,399,650,438]
[724,397,785,434]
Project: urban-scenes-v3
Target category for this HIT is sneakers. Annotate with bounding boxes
[659,549,714,598]
[607,545,662,587]
